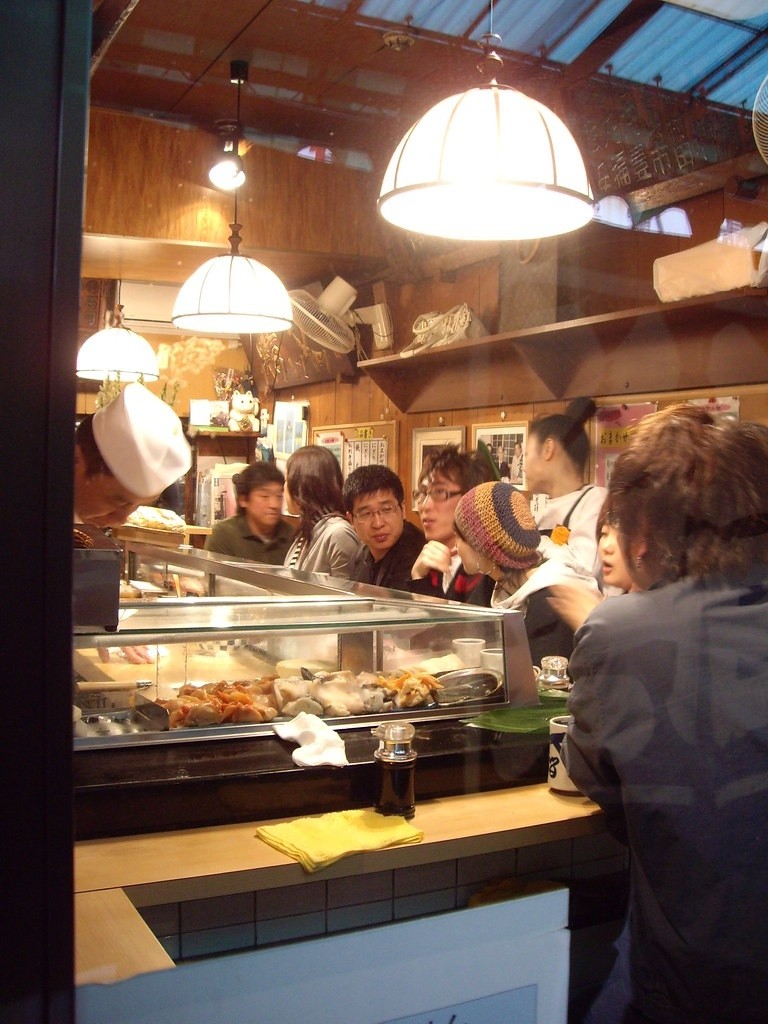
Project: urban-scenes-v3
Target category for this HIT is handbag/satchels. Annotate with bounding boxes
[400,302,491,360]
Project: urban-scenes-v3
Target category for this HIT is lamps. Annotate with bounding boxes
[171,61,295,334]
[204,118,247,193]
[374,0,596,241]
[75,279,162,384]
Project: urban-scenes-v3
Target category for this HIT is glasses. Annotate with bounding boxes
[412,487,463,504]
[352,502,398,521]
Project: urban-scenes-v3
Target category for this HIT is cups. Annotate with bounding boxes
[548,716,579,792]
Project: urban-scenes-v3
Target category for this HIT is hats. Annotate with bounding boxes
[92,382,192,498]
[453,481,544,570]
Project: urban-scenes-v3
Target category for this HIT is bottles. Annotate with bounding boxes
[371,721,418,820]
[541,655,571,692]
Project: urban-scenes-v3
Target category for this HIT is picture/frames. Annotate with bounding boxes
[471,419,531,492]
[411,425,466,511]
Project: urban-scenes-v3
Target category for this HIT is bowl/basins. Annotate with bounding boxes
[481,648,503,674]
[452,638,486,667]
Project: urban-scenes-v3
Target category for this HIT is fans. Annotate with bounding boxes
[288,275,395,355]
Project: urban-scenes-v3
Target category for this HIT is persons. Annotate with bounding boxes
[561,404,768,1024]
[510,442,523,483]
[73,381,193,663]
[209,397,646,682]
[486,444,492,454]
[495,446,510,479]
